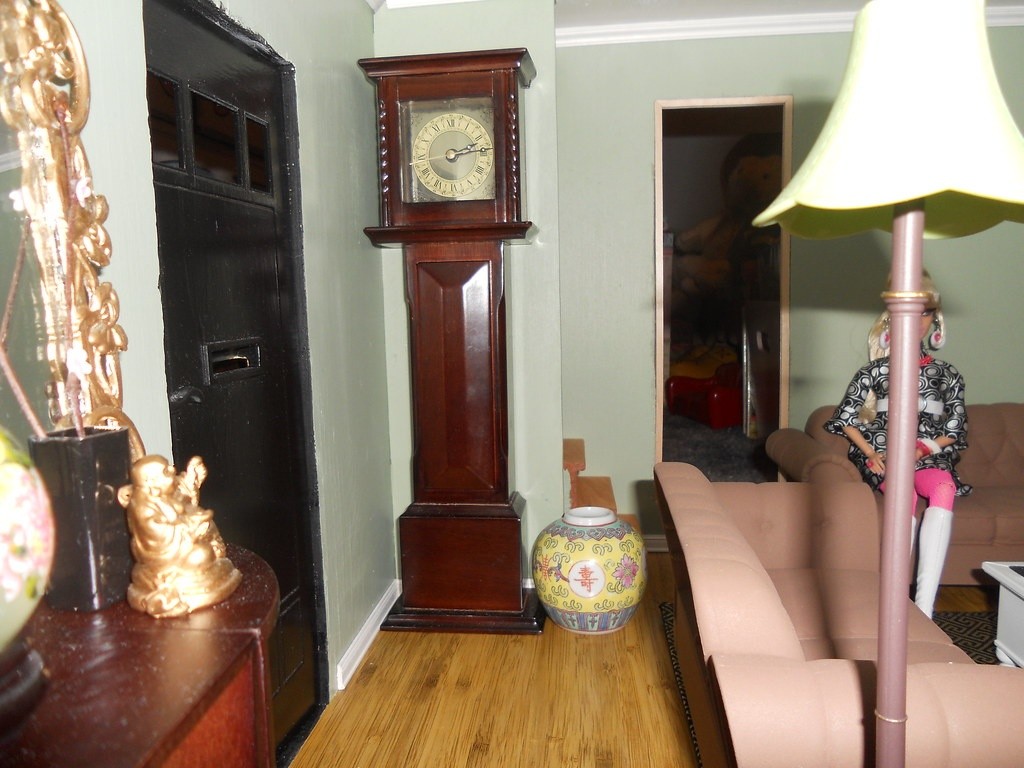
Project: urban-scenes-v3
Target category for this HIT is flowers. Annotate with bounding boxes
[0,106,100,441]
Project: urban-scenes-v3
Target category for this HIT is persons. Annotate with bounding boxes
[824,269,973,616]
[128,456,226,590]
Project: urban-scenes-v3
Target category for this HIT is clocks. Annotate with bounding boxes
[409,105,496,203]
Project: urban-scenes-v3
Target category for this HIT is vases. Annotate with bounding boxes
[27,425,137,611]
[530,507,649,636]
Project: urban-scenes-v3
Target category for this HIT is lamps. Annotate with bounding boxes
[750,0,1024,768]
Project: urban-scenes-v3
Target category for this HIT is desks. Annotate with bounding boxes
[0,541,280,767]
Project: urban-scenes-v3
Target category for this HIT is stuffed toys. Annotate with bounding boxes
[673,154,782,294]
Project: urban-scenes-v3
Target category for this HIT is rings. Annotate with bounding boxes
[867,461,873,467]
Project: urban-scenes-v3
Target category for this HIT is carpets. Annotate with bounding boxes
[659,600,1003,768]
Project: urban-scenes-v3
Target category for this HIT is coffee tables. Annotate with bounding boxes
[982,561,1024,669]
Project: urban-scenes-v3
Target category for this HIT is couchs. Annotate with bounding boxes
[765,403,1024,586]
[653,462,1024,768]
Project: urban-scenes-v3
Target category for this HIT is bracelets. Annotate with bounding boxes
[917,438,943,456]
